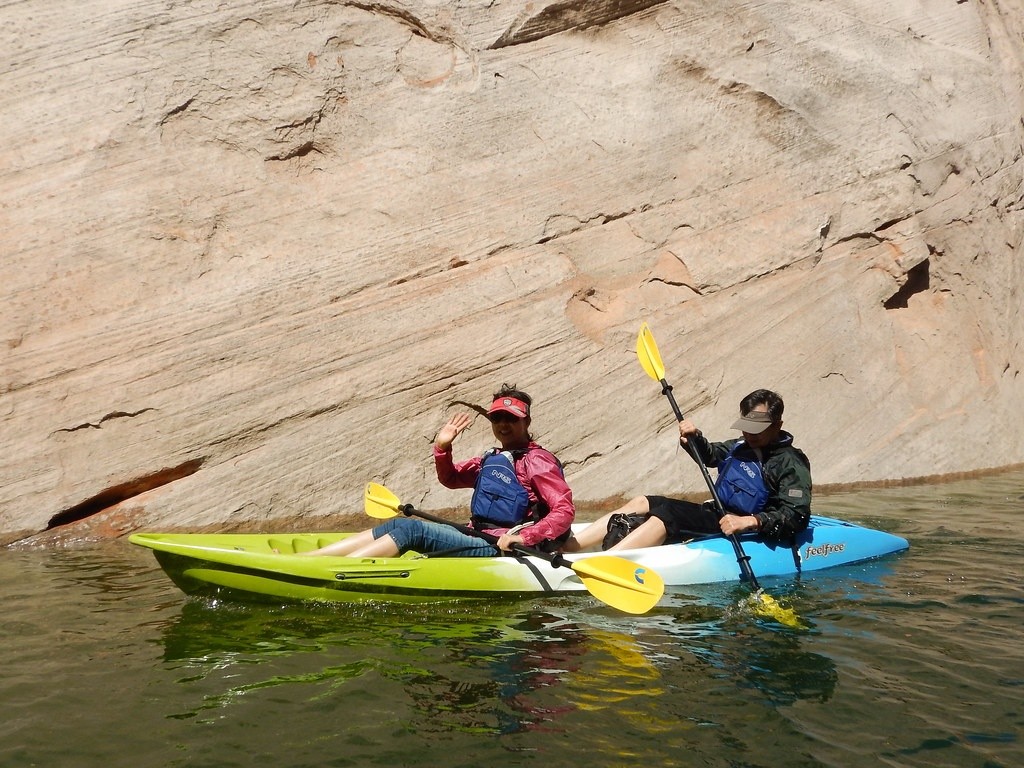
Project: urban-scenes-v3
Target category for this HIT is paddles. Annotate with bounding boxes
[634,320,803,627]
[363,480,666,616]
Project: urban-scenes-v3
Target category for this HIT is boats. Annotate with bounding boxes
[127,512,910,606]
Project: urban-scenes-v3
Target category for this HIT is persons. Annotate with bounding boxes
[559,389,812,553]
[273,383,575,559]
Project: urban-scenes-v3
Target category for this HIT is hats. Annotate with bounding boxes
[730,411,782,435]
[486,396,529,419]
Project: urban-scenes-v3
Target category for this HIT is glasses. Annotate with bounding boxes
[489,416,520,425]
